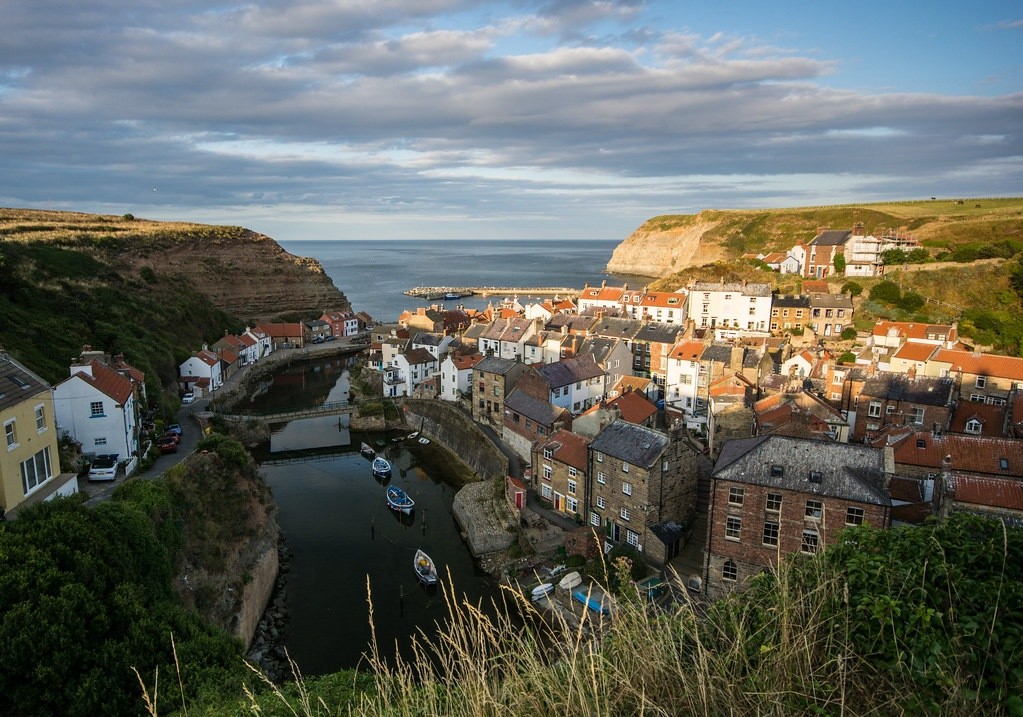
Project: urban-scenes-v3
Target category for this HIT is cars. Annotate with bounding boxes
[358,336,372,344]
[325,335,337,341]
[157,436,177,453]
[168,424,182,434]
[312,337,324,344]
[182,392,195,405]
[163,431,181,443]
[87,453,120,482]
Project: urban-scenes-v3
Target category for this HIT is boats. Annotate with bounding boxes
[391,437,405,442]
[418,438,430,444]
[386,483,416,516]
[359,441,376,455]
[559,571,583,590]
[444,291,462,300]
[407,431,418,440]
[412,547,438,586]
[531,583,556,597]
[372,455,391,479]
